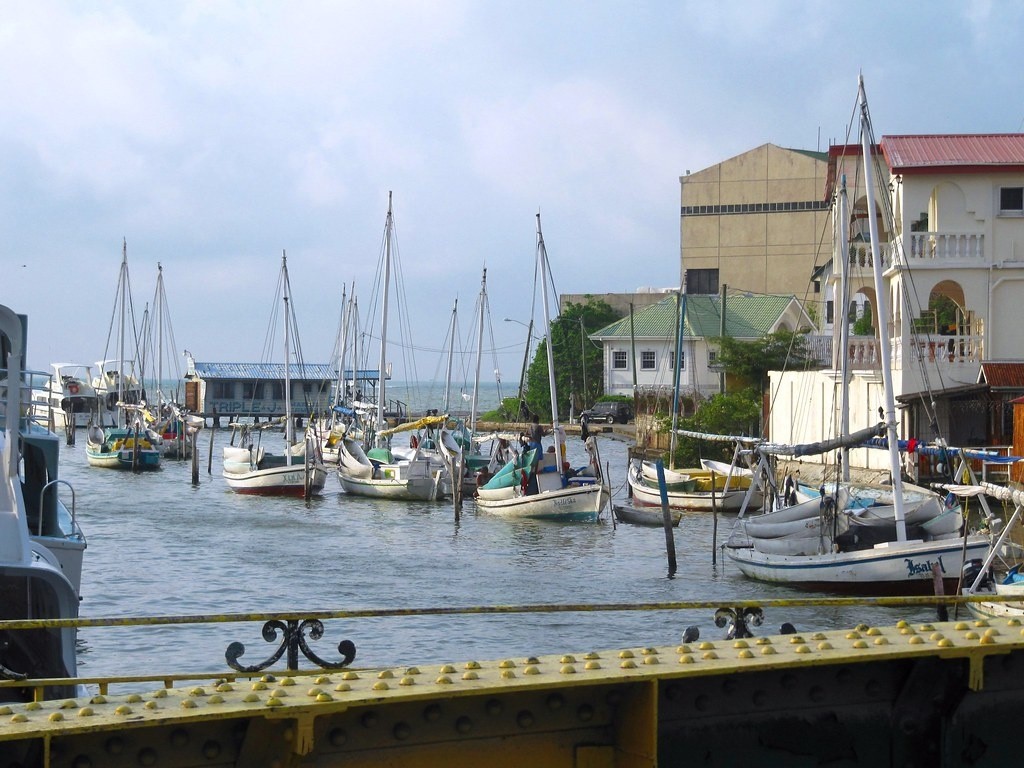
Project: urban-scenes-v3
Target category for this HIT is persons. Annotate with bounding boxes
[524,415,547,473]
[477,466,494,486]
[346,385,363,402]
[548,420,567,467]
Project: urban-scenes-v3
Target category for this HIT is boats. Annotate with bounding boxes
[92,358,148,428]
[0,303,88,703]
[30,361,103,429]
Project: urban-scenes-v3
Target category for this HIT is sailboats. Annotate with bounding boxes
[84,237,196,470]
[221,64,1023,599]
